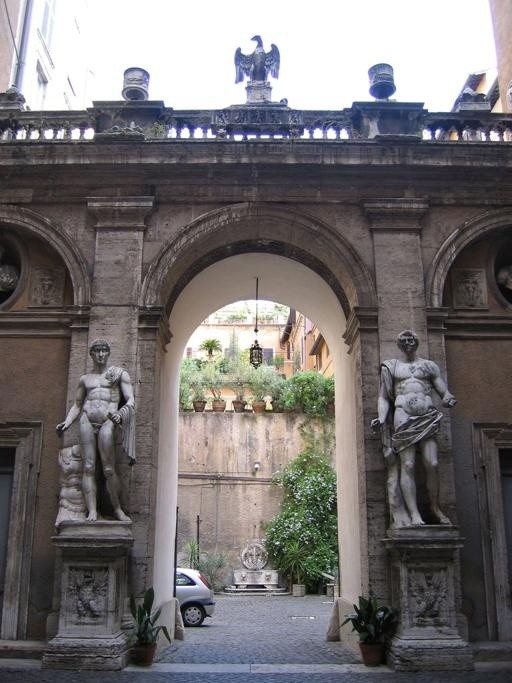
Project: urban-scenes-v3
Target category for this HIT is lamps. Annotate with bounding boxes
[248,276,264,371]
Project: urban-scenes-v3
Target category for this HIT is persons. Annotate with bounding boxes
[55,337,136,522]
[370,329,458,525]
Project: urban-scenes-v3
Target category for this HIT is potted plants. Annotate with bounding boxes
[180,357,335,416]
[336,594,400,667]
[124,587,172,667]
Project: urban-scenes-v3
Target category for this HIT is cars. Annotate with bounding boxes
[173,565,217,628]
